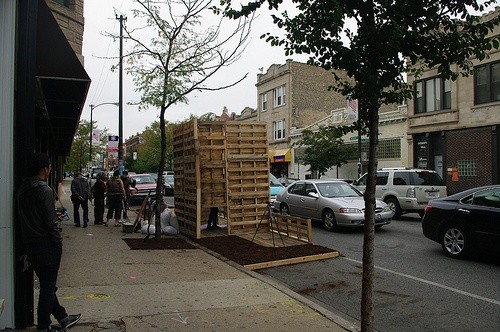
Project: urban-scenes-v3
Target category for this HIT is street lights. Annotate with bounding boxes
[90,102,119,194]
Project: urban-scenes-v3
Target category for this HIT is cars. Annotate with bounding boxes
[269,173,395,232]
[127,174,157,205]
[421,185,500,259]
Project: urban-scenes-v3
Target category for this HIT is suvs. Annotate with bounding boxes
[91,165,102,178]
[162,171,174,195]
[349,167,447,220]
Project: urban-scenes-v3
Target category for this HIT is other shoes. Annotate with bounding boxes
[115,224,121,227]
[59,313,83,331]
[74,224,80,227]
[83,223,88,228]
[123,216,128,219]
[94,221,102,225]
[102,221,106,224]
[105,224,108,227]
[37,324,67,332]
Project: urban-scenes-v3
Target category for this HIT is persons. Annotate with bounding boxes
[92,172,108,224]
[70,172,90,228]
[63,171,66,179]
[105,170,127,227]
[120,170,136,219]
[16,151,84,332]
[206,207,222,232]
